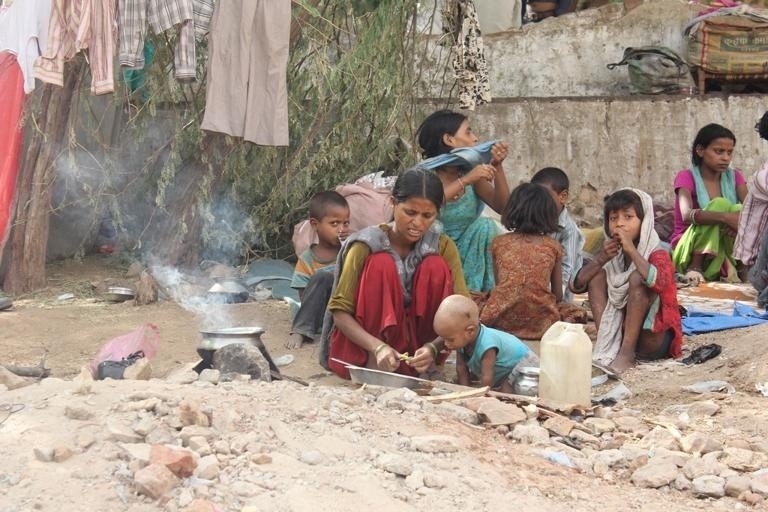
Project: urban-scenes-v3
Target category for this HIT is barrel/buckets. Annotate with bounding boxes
[537,320,595,411]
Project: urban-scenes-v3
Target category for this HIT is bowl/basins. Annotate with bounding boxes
[101,288,135,301]
[511,367,538,396]
[344,366,425,390]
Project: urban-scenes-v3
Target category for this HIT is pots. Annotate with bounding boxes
[208,277,248,304]
[195,327,265,362]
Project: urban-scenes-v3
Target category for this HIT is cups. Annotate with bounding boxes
[338,233,345,246]
[679,86,696,97]
